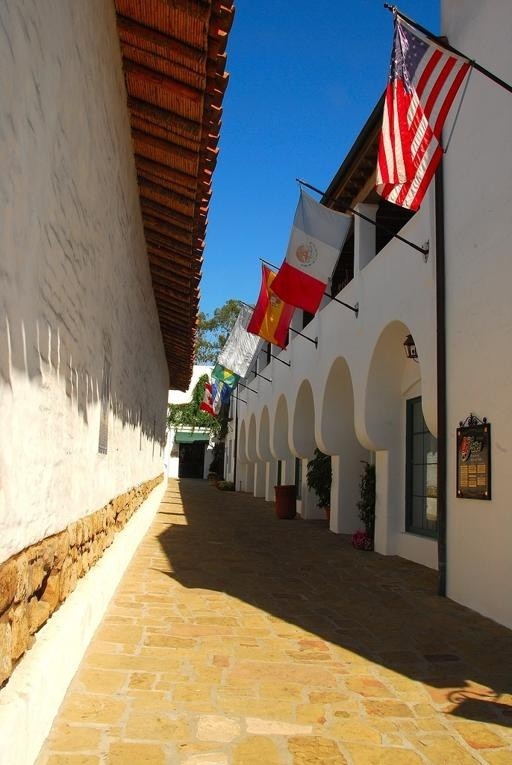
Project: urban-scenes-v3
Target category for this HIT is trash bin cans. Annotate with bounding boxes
[274,485,297,519]
[207,472,218,486]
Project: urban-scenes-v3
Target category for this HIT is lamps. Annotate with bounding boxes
[403,334,419,363]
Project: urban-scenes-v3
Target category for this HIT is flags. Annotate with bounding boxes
[374,15,472,210]
[246,266,297,349]
[217,302,266,380]
[196,362,242,419]
[270,189,355,317]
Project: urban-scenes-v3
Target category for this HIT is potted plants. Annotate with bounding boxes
[356,459,375,551]
[306,447,331,521]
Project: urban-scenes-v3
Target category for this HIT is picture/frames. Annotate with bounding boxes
[456,424,491,500]
